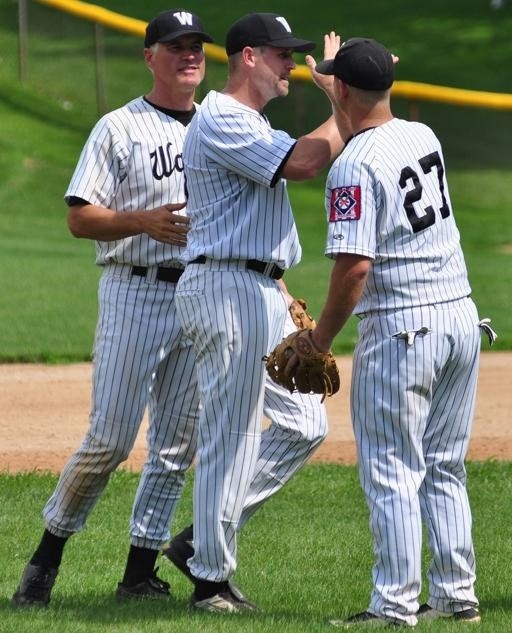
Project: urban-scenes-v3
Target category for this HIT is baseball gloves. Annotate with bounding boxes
[288,299,315,329]
[262,329,339,405]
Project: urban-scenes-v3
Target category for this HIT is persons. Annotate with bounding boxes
[9,7,218,612]
[256,35,489,627]
[171,7,403,617]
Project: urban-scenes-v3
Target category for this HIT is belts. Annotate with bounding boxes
[132,264,184,283]
[187,253,286,280]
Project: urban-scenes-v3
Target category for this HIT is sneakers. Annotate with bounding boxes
[415,602,480,623]
[330,611,414,627]
[163,523,200,581]
[190,588,257,611]
[114,576,171,599]
[12,559,59,604]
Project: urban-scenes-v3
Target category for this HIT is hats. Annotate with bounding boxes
[225,11,316,52]
[314,36,395,91]
[144,8,214,45]
